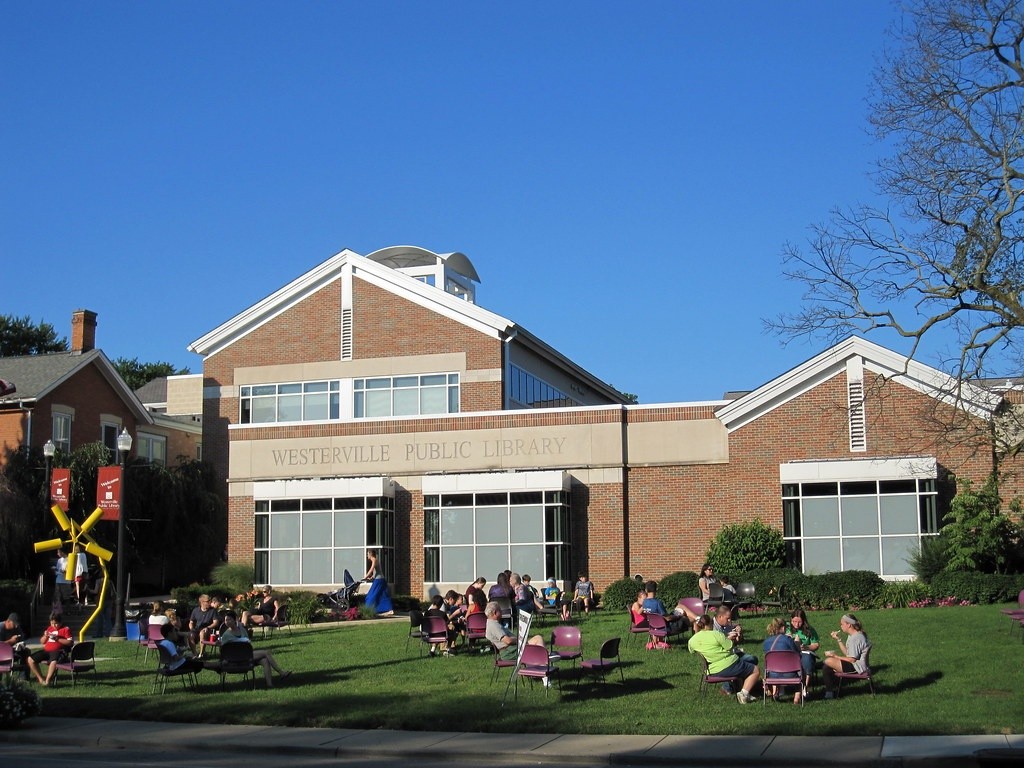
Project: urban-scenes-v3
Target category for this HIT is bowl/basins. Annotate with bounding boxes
[729,631,737,636]
[49,634,56,638]
[825,650,835,656]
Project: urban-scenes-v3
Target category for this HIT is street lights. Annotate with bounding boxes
[111,428,132,636]
[44,439,56,541]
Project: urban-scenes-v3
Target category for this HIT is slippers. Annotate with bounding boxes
[279,671,293,680]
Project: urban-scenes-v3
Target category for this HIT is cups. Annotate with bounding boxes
[210,634,216,642]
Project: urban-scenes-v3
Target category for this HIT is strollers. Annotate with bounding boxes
[317,568,371,613]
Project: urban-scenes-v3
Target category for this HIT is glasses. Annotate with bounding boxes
[262,591,267,593]
[707,569,714,571]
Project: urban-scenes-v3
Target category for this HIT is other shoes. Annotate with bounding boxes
[443,651,453,657]
[549,652,562,661]
[192,651,208,659]
[427,650,435,658]
[803,685,810,698]
[542,677,552,687]
[718,686,736,696]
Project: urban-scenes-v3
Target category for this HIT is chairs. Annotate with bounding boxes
[1,603,292,694]
[997,589,1024,638]
[405,584,874,709]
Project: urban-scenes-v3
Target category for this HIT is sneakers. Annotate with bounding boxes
[736,691,751,705]
[747,694,757,702]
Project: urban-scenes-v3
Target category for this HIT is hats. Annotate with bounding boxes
[9,613,22,625]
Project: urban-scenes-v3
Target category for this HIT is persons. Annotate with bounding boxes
[629,563,870,706]
[0,613,31,683]
[27,612,73,686]
[361,549,394,617]
[485,601,551,689]
[74,544,89,606]
[148,585,292,687]
[52,546,72,616]
[420,570,594,658]
